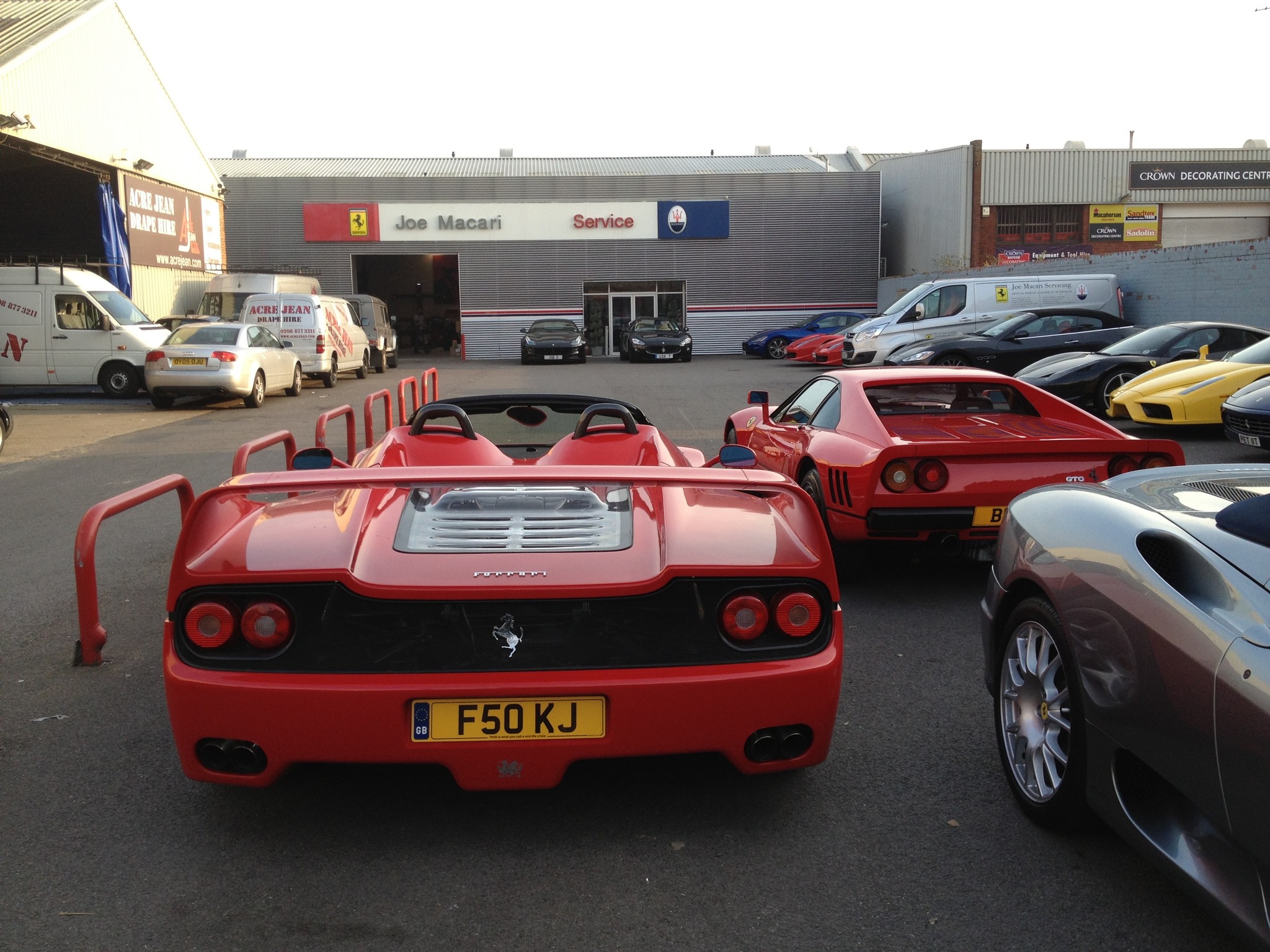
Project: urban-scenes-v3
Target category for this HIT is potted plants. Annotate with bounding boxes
[589,300,604,356]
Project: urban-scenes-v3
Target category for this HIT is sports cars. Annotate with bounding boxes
[881,306,1269,452]
[723,364,1185,570]
[741,312,870,367]
[160,391,843,794]
[979,462,1270,952]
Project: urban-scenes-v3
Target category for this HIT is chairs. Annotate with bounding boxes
[950,395,993,413]
[535,404,692,467]
[57,299,95,329]
[354,404,515,465]
[1038,320,1070,335]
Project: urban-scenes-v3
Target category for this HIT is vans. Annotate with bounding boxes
[195,272,398,387]
[1,264,174,400]
[840,275,1128,368]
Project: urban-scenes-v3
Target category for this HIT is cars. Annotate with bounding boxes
[521,320,589,364]
[601,315,631,342]
[144,315,303,408]
[619,317,693,362]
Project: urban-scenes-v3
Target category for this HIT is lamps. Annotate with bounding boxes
[134,159,154,170]
[1120,194,1132,203]
[217,188,230,195]
